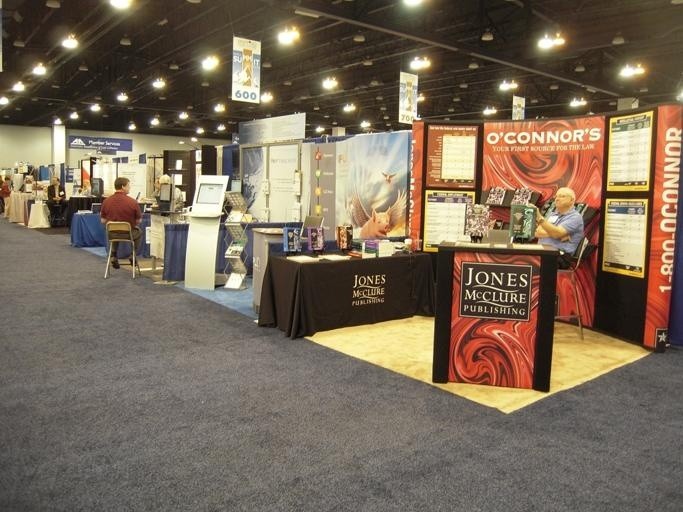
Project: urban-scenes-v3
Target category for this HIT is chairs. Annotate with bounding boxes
[104,221,142,279]
[298,215,324,239]
[554,236,588,338]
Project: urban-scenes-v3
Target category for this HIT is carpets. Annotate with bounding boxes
[173,283,261,318]
[254,316,657,412]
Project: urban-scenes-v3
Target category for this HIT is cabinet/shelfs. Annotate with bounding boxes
[163,149,191,207]
[223,191,250,290]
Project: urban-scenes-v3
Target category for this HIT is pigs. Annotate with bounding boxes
[359,205,391,239]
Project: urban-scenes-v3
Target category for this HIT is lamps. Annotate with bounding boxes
[444,29,494,119]
[168,55,330,118]
[352,30,394,132]
[44,1,61,9]
[78,56,89,71]
[11,33,25,48]
[120,29,132,46]
[530,30,649,105]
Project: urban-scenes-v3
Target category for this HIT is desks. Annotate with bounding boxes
[71,210,150,258]
[162,221,303,281]
[252,227,284,314]
[258,249,436,339]
[4,191,109,230]
[149,208,184,271]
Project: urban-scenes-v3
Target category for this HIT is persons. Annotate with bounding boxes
[0,175,35,218]
[99,177,143,269]
[150,173,183,213]
[45,176,66,221]
[526,187,586,269]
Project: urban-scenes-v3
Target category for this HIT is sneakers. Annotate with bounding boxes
[111,257,119,269]
[128,255,136,267]
